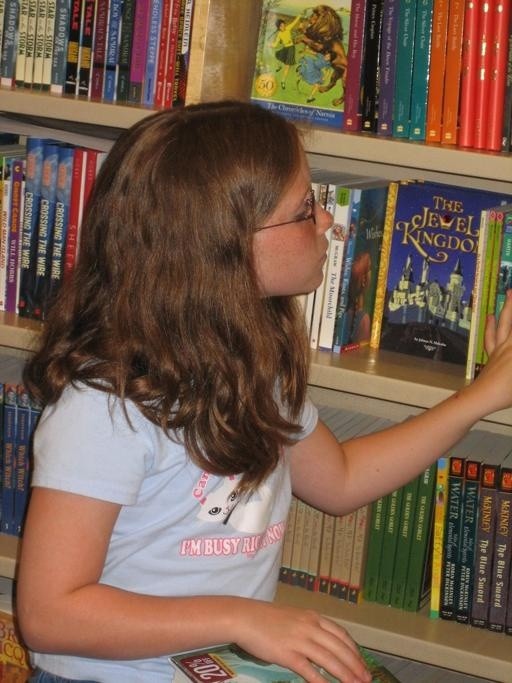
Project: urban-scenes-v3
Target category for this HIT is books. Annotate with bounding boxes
[2,355,55,536]
[251,1,512,155]
[0,135,110,322]
[0,0,194,108]
[290,168,512,381]
[278,405,512,636]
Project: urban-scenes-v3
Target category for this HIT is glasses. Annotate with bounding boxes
[254,190,315,232]
